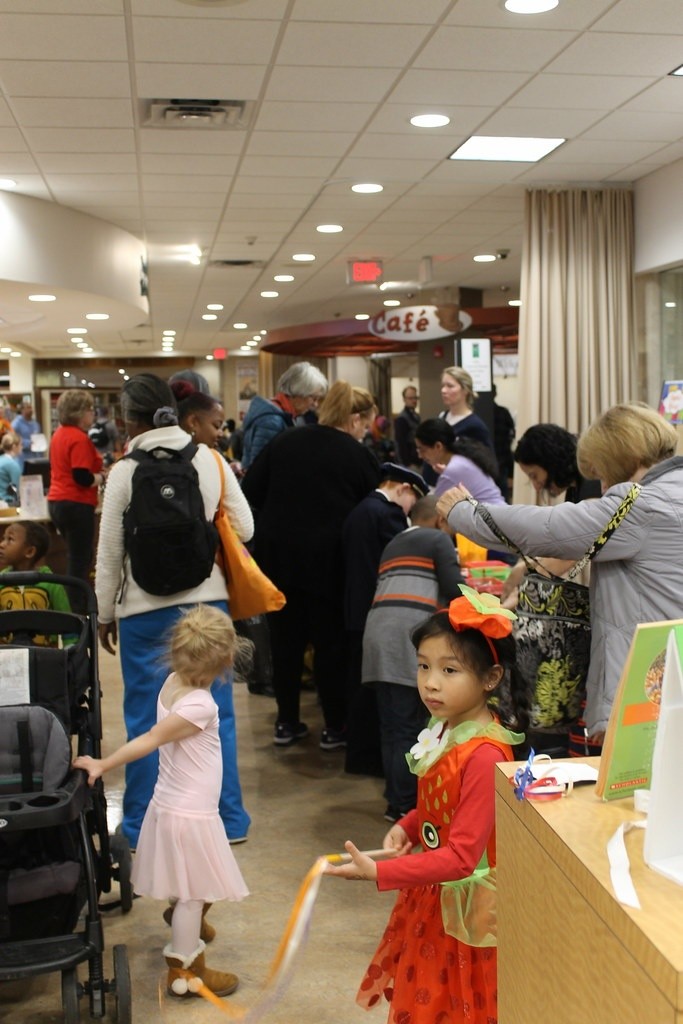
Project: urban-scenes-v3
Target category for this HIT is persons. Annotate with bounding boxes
[321,611,532,1024]
[0,360,601,852]
[435,401,683,756]
[70,603,254,997]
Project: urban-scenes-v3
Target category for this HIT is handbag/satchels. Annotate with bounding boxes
[484,570,592,732]
[209,447,287,621]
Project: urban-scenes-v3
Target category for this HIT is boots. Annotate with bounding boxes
[162,939,240,998]
[161,897,215,942]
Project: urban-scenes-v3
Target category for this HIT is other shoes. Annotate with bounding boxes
[227,834,249,845]
[272,721,309,743]
[114,822,138,852]
[346,762,386,779]
[383,801,413,821]
[320,728,349,748]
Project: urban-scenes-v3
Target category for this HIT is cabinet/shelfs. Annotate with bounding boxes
[40,387,128,448]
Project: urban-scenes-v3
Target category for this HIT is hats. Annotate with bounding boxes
[379,461,430,499]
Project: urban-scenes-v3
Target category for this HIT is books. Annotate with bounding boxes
[594,619,683,801]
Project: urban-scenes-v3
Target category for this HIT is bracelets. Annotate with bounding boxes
[99,473,105,482]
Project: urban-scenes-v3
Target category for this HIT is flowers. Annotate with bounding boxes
[449,583,518,638]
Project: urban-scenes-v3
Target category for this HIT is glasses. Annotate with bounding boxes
[85,404,95,413]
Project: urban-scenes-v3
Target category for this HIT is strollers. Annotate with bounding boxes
[0,569,133,1024]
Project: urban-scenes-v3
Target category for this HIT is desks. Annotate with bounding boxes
[494,757,683,1024]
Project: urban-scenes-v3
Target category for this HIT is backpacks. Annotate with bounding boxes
[118,442,219,596]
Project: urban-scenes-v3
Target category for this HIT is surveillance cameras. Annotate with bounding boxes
[496,249,510,260]
